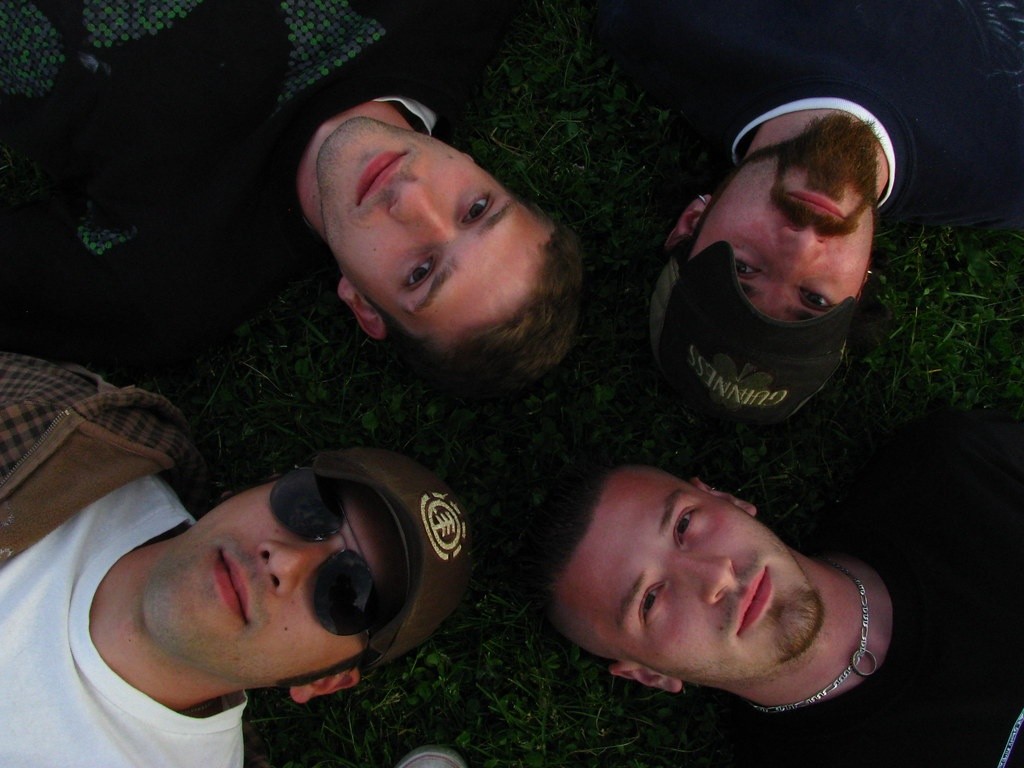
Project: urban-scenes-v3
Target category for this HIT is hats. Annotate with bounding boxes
[649,240,857,424]
[289,446,470,676]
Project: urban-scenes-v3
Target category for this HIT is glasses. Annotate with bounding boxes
[270,466,393,654]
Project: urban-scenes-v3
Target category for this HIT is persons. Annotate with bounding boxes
[591,0,1024,423]
[0,350,469,768]
[509,409,1024,768]
[0,0,580,402]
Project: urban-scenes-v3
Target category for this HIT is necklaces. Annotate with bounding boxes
[749,554,877,712]
